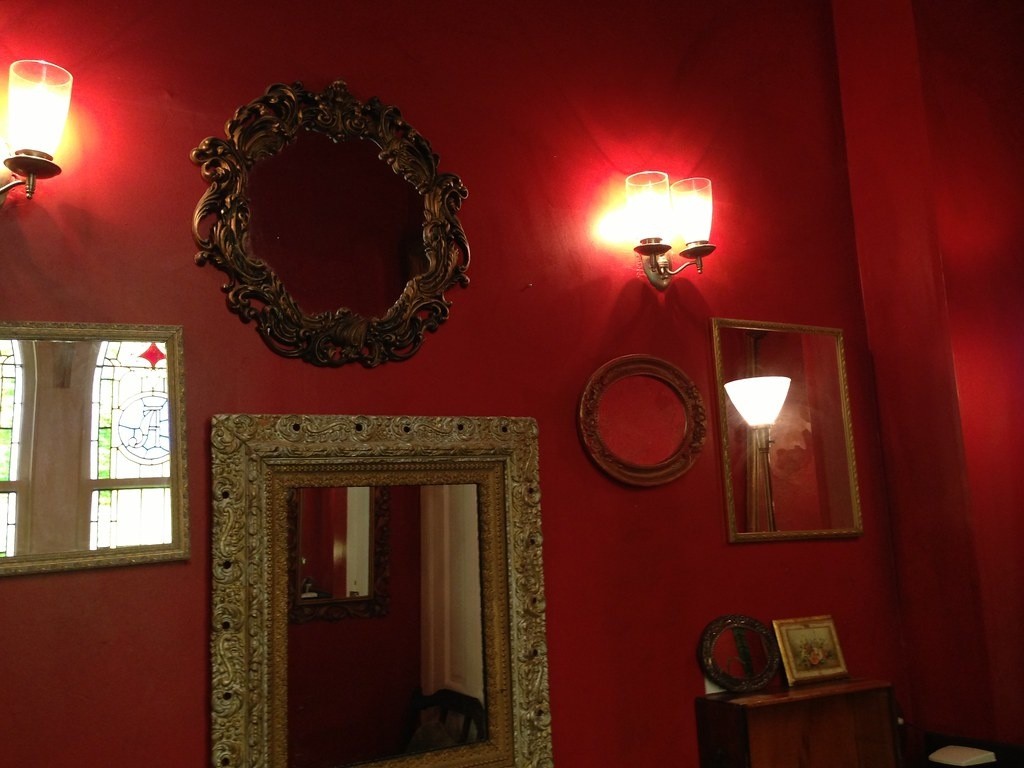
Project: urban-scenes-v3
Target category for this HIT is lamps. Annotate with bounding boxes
[0,57,74,206]
[627,172,716,289]
[724,376,792,533]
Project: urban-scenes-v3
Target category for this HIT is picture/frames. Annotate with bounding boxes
[772,614,848,689]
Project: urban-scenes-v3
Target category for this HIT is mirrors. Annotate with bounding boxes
[577,354,706,487]
[190,80,472,369]
[209,413,554,768]
[0,321,191,576]
[709,318,865,544]
[288,487,391,621]
[699,614,780,692]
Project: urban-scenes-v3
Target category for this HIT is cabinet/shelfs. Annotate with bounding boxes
[696,680,903,768]
